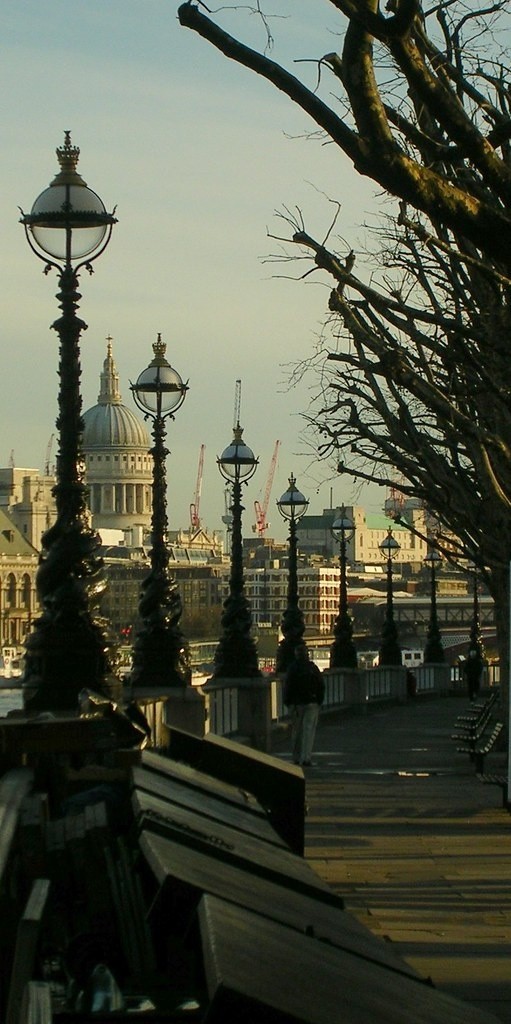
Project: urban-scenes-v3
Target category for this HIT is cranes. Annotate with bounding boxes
[255,440,281,536]
[179,442,209,538]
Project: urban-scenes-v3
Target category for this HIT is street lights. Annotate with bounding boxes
[272,472,313,671]
[467,559,484,648]
[5,129,155,756]
[421,545,447,665]
[125,331,194,689]
[210,380,264,679]
[375,524,407,667]
[328,501,362,669]
